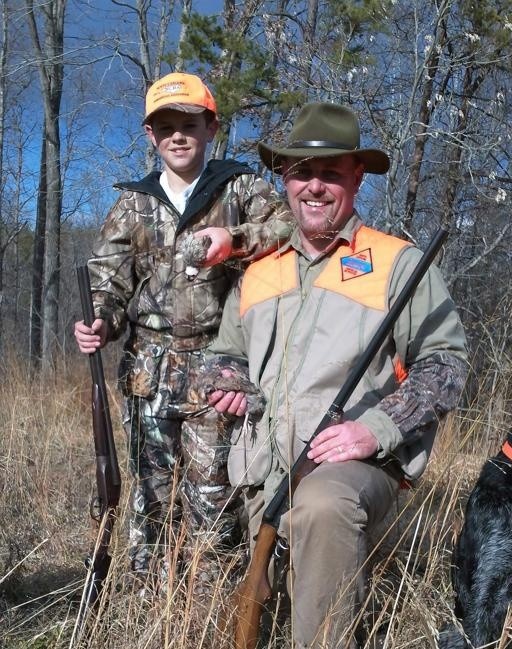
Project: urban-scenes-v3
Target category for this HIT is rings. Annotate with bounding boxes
[214,255,221,260]
[337,446,343,454]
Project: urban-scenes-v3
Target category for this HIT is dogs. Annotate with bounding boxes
[438,427,512,649]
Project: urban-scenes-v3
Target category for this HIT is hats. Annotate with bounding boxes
[257,101,390,175]
[140,71,218,127]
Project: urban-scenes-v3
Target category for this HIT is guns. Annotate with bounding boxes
[73,268,124,615]
[234,223,453,649]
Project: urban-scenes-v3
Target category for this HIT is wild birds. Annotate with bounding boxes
[182,232,212,282]
[167,365,266,437]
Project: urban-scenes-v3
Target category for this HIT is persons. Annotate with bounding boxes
[73,73,298,622]
[203,101,480,649]
